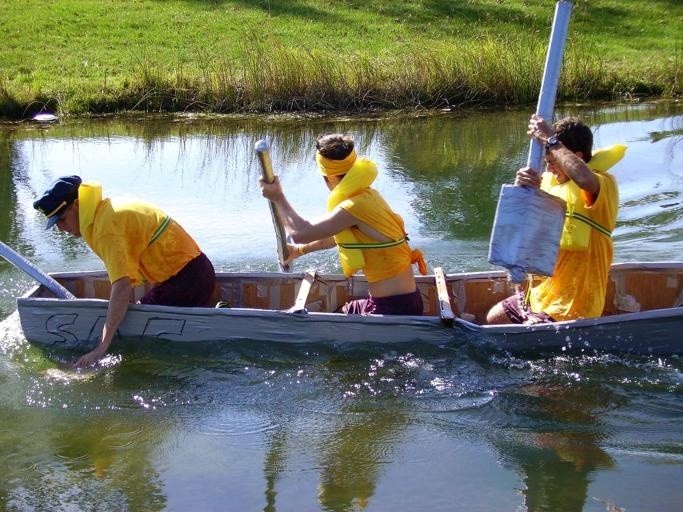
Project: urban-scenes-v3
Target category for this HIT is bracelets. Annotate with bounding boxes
[298,245,303,255]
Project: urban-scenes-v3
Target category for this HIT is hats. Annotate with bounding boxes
[30,174,82,233]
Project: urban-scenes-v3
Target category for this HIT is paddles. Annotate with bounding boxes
[487,0,573,284]
[0,240,79,300]
[253,139,294,273]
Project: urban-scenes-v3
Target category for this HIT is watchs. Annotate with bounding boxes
[543,137,560,149]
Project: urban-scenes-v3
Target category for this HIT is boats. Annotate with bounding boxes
[16,259,683,358]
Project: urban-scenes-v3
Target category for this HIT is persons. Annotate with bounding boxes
[259,135,428,315]
[487,116,628,324]
[33,175,216,366]
